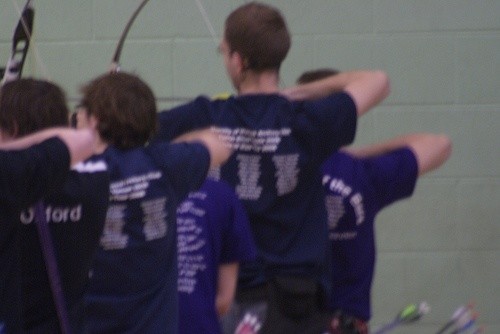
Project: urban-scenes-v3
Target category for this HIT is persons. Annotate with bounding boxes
[40,71,237,334]
[156,3,390,334]
[297,68,453,334]
[0,77,255,334]
